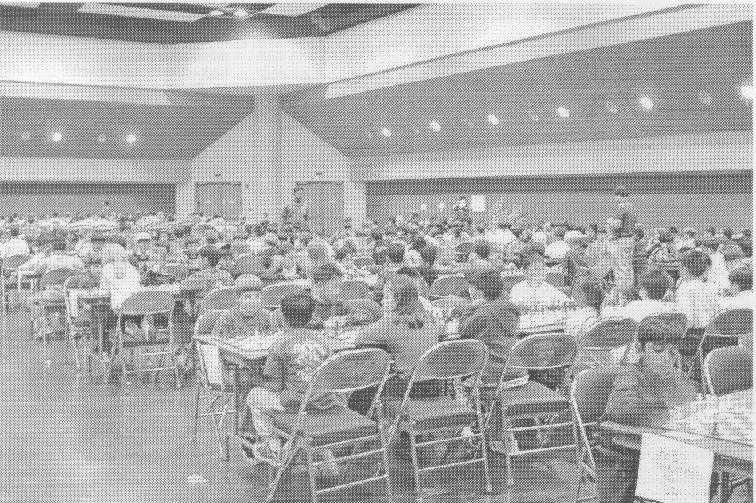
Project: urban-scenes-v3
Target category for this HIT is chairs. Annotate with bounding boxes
[2,226,753,344]
[569,315,641,483]
[703,345,753,395]
[184,306,259,457]
[262,348,396,502]
[697,306,752,371]
[633,309,687,356]
[61,274,97,370]
[375,338,492,495]
[564,365,666,502]
[105,289,182,390]
[473,332,586,487]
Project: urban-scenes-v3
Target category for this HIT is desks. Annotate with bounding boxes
[596,389,753,502]
[197,303,604,469]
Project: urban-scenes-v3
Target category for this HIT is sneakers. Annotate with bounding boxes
[38,327,54,338]
[463,427,475,454]
[316,465,338,479]
[254,442,284,467]
[490,440,519,458]
[242,437,256,465]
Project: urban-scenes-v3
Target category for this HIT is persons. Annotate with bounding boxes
[0,186,754,503]
